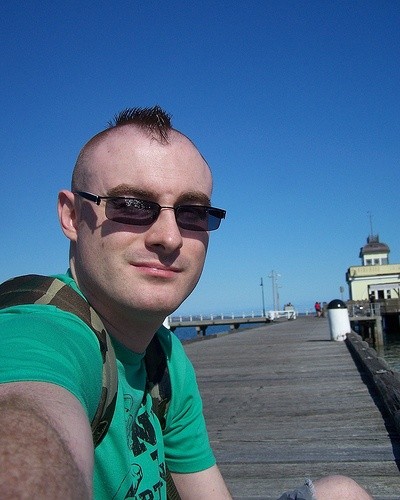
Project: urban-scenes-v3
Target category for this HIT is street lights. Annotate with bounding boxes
[268,270,281,310]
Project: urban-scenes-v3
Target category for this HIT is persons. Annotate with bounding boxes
[315,301,322,318]
[0,105,374,500]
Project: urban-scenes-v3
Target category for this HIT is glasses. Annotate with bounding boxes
[69,189,226,231]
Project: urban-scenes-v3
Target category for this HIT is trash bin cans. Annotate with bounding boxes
[327,299,351,341]
